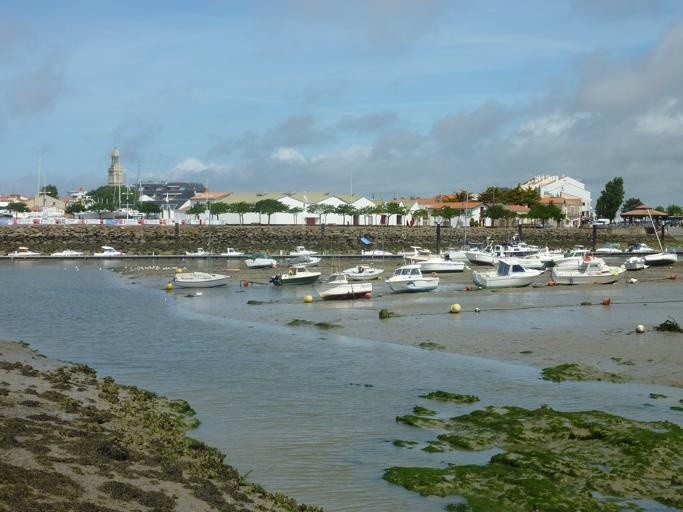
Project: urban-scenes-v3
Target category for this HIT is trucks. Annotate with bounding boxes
[593,219,610,225]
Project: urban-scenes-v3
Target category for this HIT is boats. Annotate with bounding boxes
[244,246,320,286]
[174,271,230,287]
[8,245,125,258]
[185,247,243,258]
